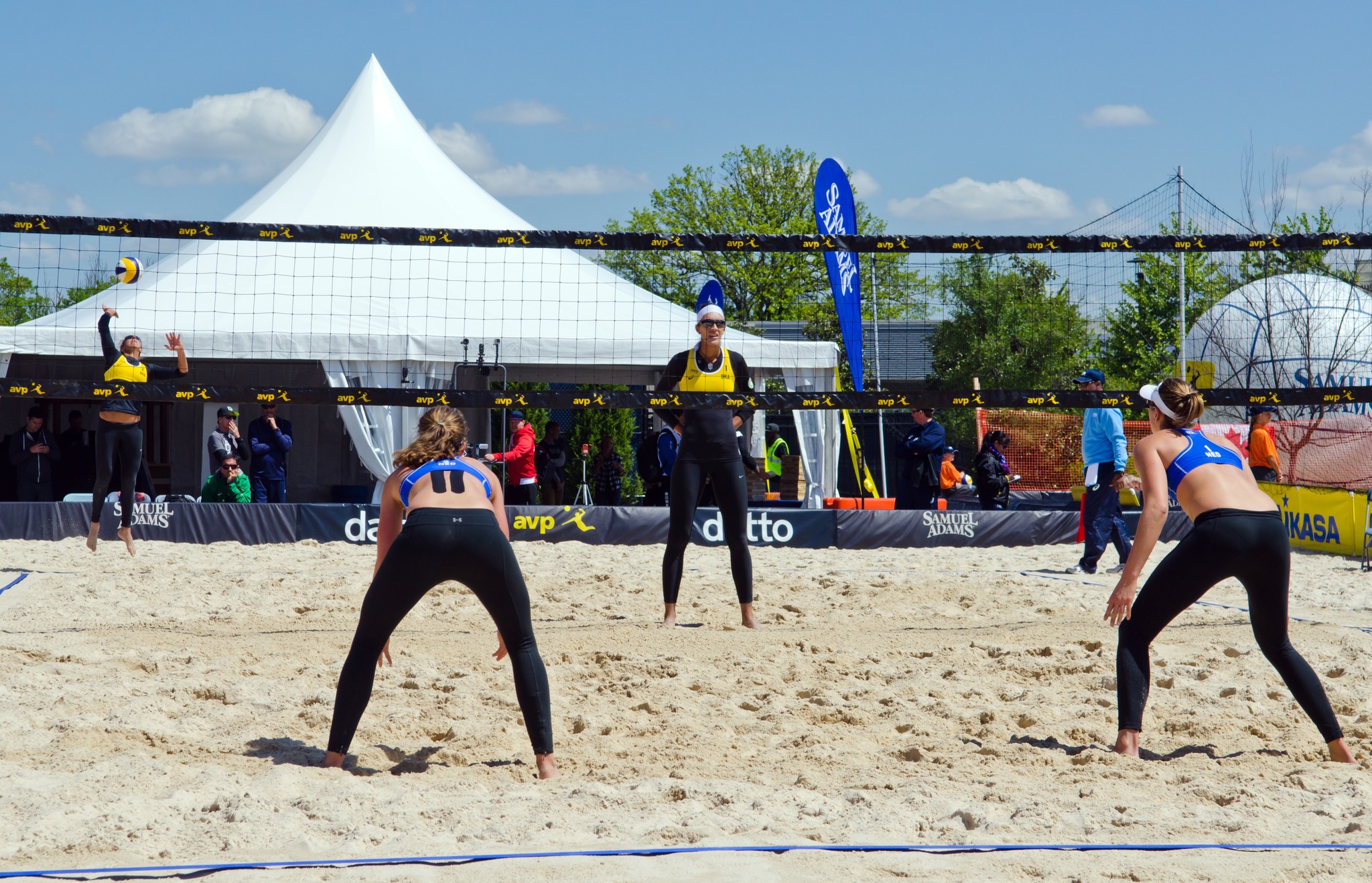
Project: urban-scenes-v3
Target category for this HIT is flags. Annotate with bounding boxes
[1201,425,1276,470]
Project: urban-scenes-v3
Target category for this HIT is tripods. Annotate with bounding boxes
[574,459,594,506]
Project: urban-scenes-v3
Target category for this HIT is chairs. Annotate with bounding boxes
[1361,487,1372,571]
[62,491,202,502]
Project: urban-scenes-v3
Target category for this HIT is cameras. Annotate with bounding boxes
[582,444,593,454]
[475,444,488,460]
[467,443,473,457]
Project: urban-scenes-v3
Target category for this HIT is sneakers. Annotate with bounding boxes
[1065,564,1096,575]
[1105,563,1142,574]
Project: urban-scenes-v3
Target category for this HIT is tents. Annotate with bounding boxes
[0,48,843,510]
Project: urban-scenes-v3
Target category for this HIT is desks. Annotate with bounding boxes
[747,500,803,508]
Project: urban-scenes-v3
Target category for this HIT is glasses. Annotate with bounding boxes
[222,464,237,470]
[699,319,725,329]
[261,404,276,410]
[999,443,1008,450]
[911,410,919,415]
[124,335,142,342]
[224,414,237,420]
[1079,381,1095,387]
[508,414,521,418]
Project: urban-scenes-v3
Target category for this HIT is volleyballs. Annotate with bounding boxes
[958,474,972,485]
[115,257,144,284]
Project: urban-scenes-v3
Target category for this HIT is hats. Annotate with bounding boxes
[217,406,239,417]
[506,411,524,422]
[1139,384,1178,419]
[943,445,958,454]
[1072,368,1105,385]
[765,423,779,432]
[1250,406,1277,416]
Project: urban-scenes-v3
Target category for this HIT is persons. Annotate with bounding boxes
[323,406,556,783]
[1106,377,1358,765]
[0,302,1284,632]
